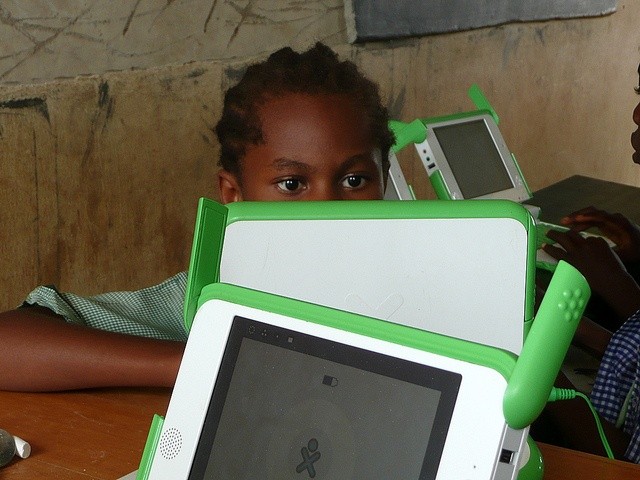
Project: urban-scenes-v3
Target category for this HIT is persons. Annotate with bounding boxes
[541,60,639,466]
[1,42,399,393]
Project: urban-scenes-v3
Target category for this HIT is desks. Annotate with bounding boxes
[528,172,640,252]
[0,387,640,480]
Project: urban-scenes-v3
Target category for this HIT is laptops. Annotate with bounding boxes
[127,196,594,480]
[376,82,618,277]
[218,198,546,475]
